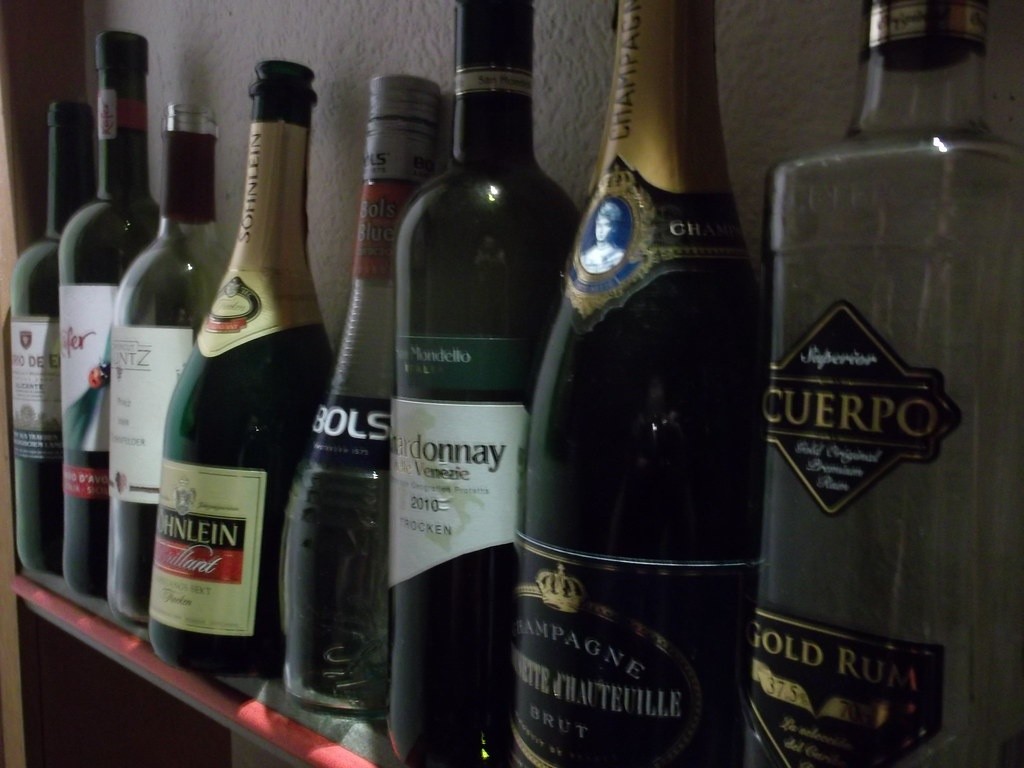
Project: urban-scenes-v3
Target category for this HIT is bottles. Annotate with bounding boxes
[60,28,160,598]
[146,58,334,676]
[276,73,448,714]
[105,98,230,624]
[509,1,765,768]
[383,0,579,768]
[743,0,1024,768]
[11,97,99,574]
